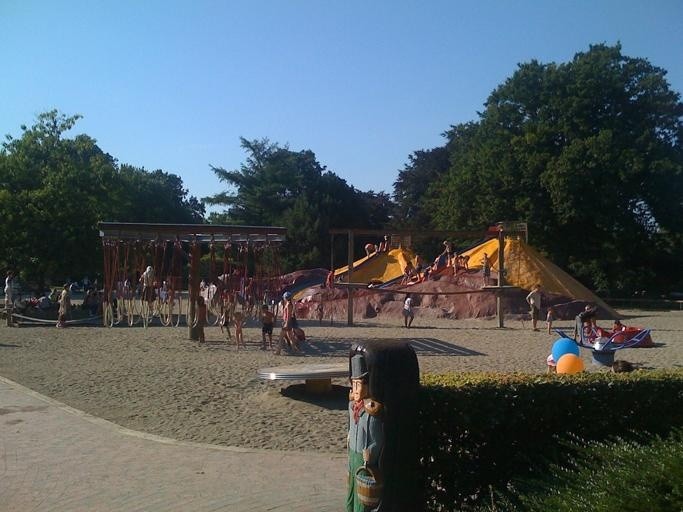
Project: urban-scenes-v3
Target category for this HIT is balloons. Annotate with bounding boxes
[553,338,579,363]
[556,353,584,374]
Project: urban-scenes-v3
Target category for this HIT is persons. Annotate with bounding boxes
[546,307,554,336]
[315,301,324,324]
[573,303,625,345]
[402,293,414,328]
[443,302,456,319]
[612,360,632,373]
[325,270,335,290]
[480,253,493,287]
[547,355,556,373]
[364,233,470,286]
[526,284,541,332]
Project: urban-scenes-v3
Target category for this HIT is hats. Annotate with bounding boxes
[281,291,292,298]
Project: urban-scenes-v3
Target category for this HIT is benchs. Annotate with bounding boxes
[259,363,350,394]
